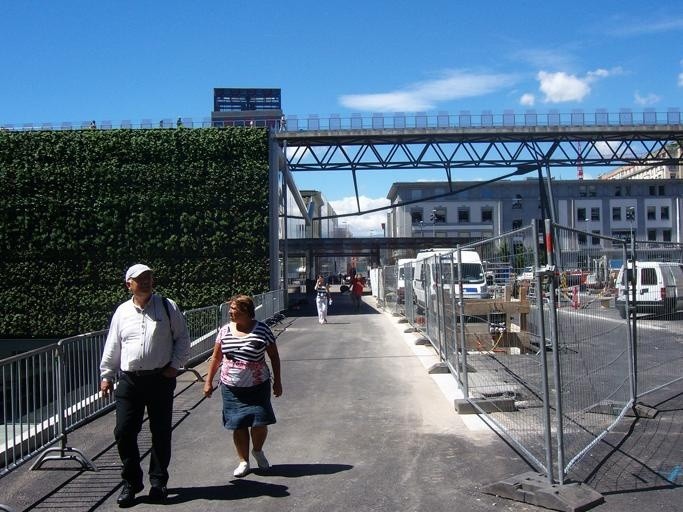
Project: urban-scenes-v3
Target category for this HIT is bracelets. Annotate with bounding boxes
[328,297,331,298]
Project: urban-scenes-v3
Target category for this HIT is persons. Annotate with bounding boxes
[348,273,365,313]
[99,263,191,506]
[314,276,332,324]
[201,293,282,478]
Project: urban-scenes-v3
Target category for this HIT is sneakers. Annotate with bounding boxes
[234,461,250,477]
[252,448,269,471]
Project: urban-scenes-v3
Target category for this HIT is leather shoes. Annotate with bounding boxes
[149,484,168,497]
[117,482,144,504]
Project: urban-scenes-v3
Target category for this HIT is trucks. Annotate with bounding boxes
[521,266,534,278]
[612,259,683,320]
[391,246,494,322]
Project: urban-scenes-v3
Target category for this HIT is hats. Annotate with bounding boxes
[125,263,153,283]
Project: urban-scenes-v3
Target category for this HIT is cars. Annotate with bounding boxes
[328,272,366,285]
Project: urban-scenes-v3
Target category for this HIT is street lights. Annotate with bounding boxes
[584,218,594,261]
[430,209,438,235]
[419,220,425,237]
[629,205,639,259]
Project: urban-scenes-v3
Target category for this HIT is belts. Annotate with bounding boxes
[136,370,155,377]
